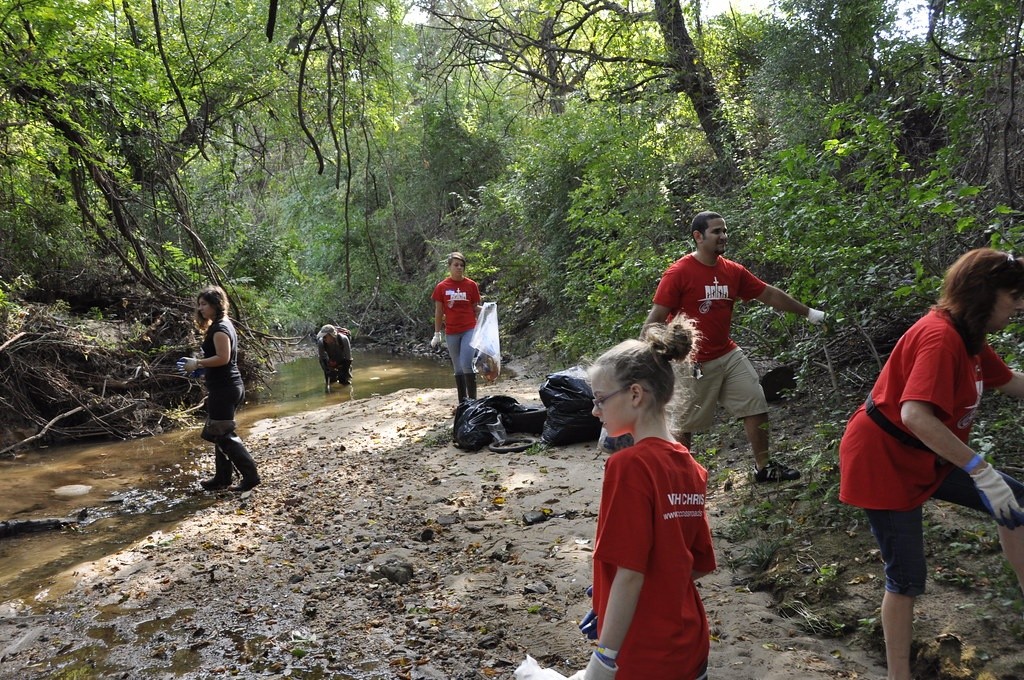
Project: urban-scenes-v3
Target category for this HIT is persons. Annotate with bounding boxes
[177,285,247,491]
[637,209,834,483]
[839,249,1024,680]
[577,306,717,680]
[430,251,494,414]
[317,324,354,385]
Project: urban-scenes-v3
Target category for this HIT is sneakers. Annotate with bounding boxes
[754,460,800,483]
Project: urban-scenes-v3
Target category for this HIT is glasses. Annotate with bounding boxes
[593,384,649,411]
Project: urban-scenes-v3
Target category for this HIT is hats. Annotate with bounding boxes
[317,324,337,338]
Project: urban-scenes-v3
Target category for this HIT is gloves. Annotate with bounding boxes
[806,307,833,326]
[583,652,618,680]
[579,586,598,639]
[176,357,198,376]
[430,332,441,348]
[970,462,1024,530]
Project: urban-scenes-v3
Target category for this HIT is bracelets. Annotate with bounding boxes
[962,452,983,473]
[596,643,618,659]
